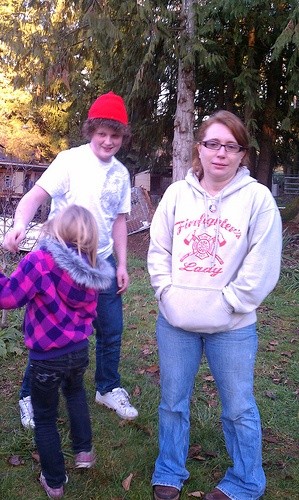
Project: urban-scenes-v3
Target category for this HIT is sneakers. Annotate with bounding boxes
[18,396,35,429]
[95,387,138,421]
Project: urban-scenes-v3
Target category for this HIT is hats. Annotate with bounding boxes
[85,91,129,127]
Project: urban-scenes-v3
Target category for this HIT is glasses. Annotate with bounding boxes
[199,140,248,154]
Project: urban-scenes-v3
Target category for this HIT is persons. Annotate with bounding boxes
[0,206,115,500]
[3,92,138,425]
[147,109,282,500]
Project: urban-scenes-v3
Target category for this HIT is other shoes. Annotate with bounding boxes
[153,484,180,500]
[39,471,65,500]
[203,487,234,500]
[73,448,95,468]
